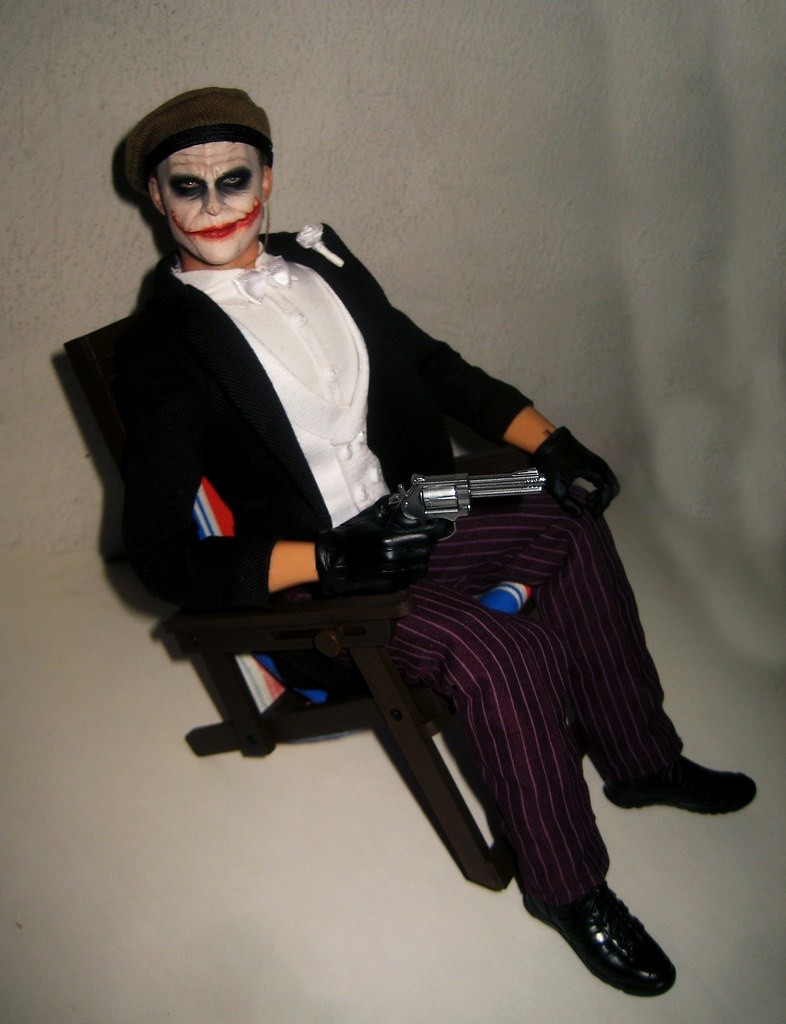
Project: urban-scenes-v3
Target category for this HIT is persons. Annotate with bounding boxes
[121,87,758,998]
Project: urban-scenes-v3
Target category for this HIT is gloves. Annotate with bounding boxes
[316,494,453,593]
[534,427,620,519]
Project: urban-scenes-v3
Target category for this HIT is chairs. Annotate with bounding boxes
[63,309,615,891]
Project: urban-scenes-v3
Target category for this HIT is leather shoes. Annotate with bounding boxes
[604,756,756,815]
[523,880,676,996]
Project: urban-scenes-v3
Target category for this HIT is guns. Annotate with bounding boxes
[375,465,547,543]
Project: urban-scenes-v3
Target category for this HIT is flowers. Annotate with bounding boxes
[296,222,346,268]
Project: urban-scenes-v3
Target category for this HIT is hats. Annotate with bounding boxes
[125,88,273,196]
